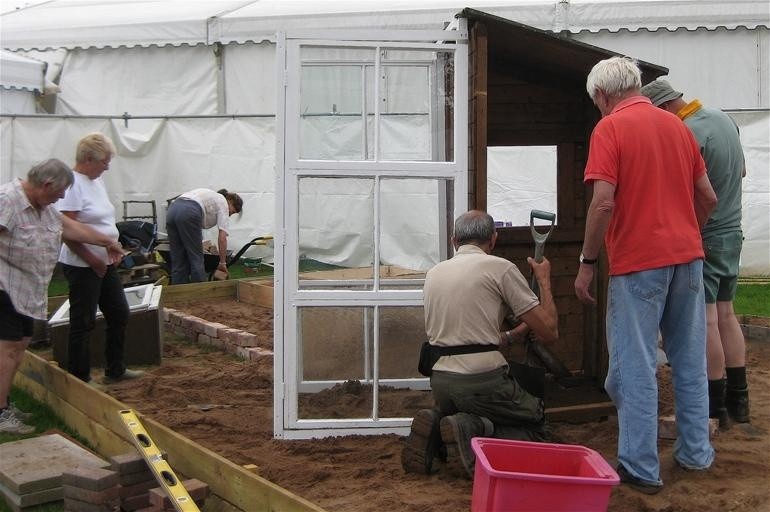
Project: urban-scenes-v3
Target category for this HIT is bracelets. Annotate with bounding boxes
[218,262,227,264]
[506,331,513,345]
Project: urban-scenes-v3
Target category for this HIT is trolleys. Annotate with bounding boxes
[155,235,273,286]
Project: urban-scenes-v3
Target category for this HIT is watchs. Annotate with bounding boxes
[578,253,597,265]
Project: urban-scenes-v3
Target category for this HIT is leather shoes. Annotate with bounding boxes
[401,408,484,481]
[708,389,750,428]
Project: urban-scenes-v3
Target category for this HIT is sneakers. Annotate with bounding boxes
[0,405,36,434]
[85,370,143,393]
[617,463,664,494]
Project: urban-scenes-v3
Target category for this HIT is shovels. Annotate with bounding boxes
[504,210,556,400]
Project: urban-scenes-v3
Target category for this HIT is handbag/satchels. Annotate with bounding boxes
[418,343,440,378]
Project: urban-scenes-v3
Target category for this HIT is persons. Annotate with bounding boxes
[402,210,559,482]
[642,80,750,431]
[573,56,717,496]
[0,158,126,435]
[166,188,243,285]
[55,133,144,390]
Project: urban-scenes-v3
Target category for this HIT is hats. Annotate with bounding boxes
[641,79,683,107]
